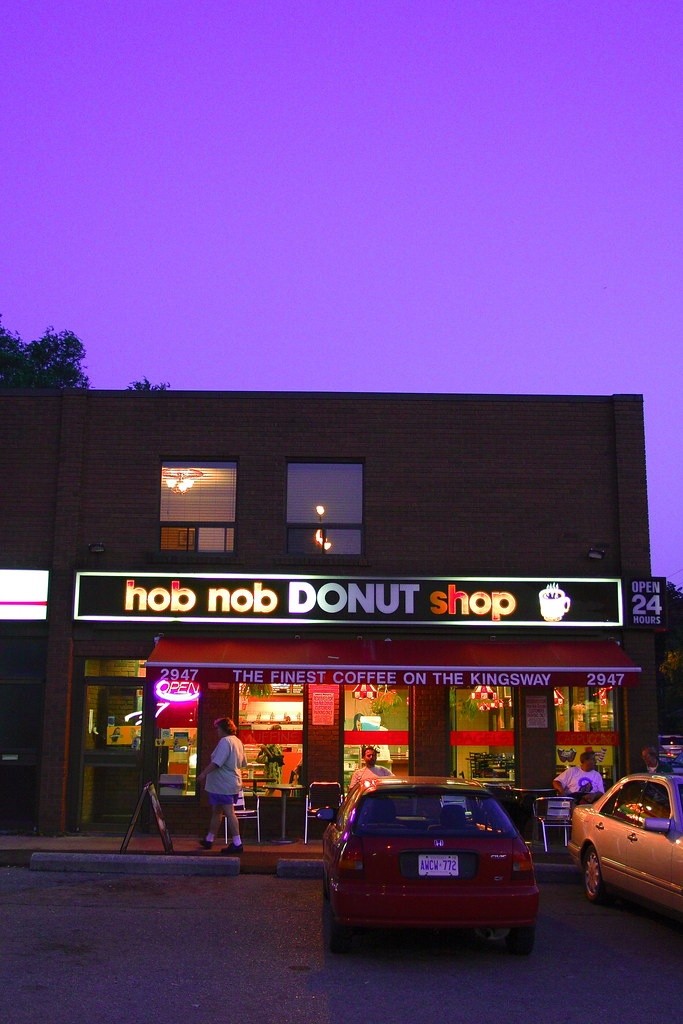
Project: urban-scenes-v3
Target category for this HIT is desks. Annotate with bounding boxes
[260,783,304,843]
[513,787,555,846]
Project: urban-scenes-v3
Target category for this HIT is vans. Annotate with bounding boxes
[659,736,683,763]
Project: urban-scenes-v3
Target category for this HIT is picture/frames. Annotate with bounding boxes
[179,529,195,546]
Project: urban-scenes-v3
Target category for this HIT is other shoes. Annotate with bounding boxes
[199,837,213,848]
[221,842,244,852]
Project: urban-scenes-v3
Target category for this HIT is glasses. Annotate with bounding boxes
[641,754,650,760]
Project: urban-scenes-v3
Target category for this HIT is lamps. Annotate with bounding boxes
[161,464,205,493]
[88,542,105,553]
[588,549,604,559]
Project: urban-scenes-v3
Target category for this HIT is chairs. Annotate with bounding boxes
[360,799,410,829]
[222,788,261,843]
[305,781,345,844]
[531,796,576,852]
[435,805,470,831]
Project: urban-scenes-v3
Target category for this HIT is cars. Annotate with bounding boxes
[315,775,541,956]
[567,771,683,924]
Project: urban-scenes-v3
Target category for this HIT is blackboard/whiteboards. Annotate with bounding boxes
[122,782,172,849]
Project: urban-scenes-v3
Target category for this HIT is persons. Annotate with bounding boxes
[289,757,303,785]
[255,725,285,796]
[348,746,397,794]
[632,744,675,774]
[352,713,364,731]
[196,716,248,854]
[553,750,605,820]
